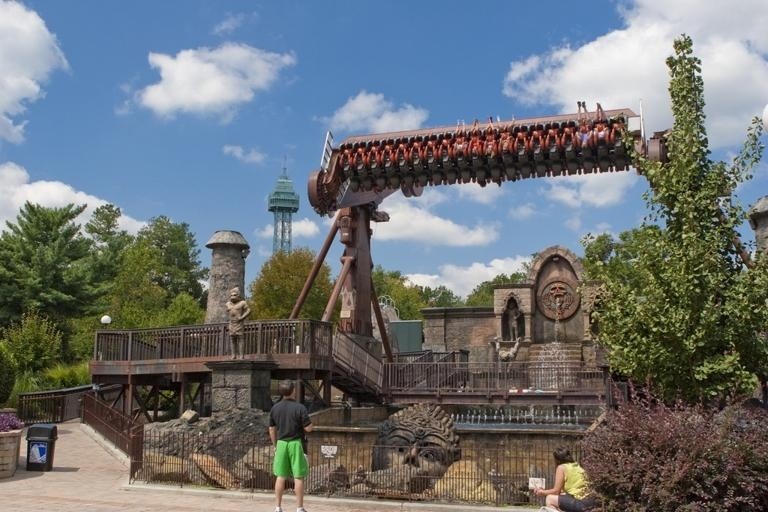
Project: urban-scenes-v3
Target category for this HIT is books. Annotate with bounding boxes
[527,476,546,491]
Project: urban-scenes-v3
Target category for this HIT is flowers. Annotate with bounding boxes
[0,412,26,432]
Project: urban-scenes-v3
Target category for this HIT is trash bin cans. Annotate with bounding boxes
[26,424,58,471]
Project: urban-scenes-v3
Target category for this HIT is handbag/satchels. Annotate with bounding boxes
[528,477,546,490]
[301,434,309,455]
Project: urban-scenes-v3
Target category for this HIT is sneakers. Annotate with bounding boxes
[297,508,307,512]
[274,507,282,512]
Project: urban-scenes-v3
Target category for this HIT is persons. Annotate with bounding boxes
[484,115,497,157]
[268,379,313,512]
[595,101,610,143]
[606,114,627,149]
[575,100,594,152]
[470,117,482,157]
[506,302,522,342]
[450,118,467,160]
[535,444,594,511]
[224,286,251,360]
[372,402,461,489]
[495,114,517,155]
